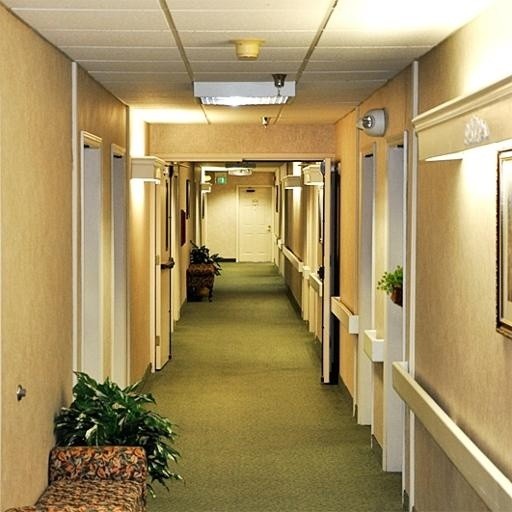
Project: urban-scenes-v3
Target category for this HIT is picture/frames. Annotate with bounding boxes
[496,151,512,342]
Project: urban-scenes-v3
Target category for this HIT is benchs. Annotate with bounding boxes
[5,445,149,511]
[187,263,215,302]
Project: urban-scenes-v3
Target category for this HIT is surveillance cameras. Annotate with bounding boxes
[361,107,387,137]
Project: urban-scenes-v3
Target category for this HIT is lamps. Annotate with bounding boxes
[281,164,324,189]
[130,155,167,182]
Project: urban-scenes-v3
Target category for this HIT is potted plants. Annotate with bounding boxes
[376,264,403,306]
